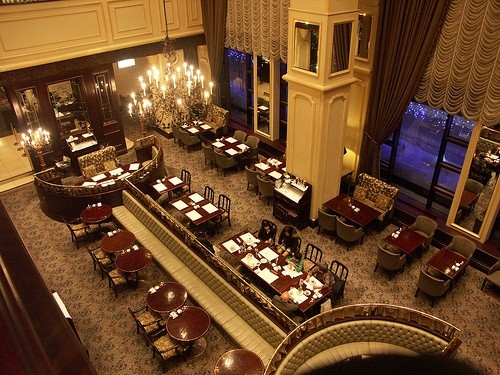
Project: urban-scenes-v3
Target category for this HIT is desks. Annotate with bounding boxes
[460,190,478,219]
[79,126,474,375]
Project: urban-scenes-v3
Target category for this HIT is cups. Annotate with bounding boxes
[298,279,322,302]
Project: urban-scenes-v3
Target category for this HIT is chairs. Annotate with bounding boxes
[181,100,500,317]
[64,180,190,374]
[456,179,483,222]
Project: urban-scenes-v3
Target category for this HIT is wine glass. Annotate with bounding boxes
[255,249,258,257]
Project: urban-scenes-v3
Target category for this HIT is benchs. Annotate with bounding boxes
[77,146,120,179]
[200,104,228,135]
[352,173,397,229]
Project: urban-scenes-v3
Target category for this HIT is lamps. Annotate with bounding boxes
[18,127,50,170]
[128,0,213,129]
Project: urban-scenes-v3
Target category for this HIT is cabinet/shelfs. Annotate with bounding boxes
[270,174,312,231]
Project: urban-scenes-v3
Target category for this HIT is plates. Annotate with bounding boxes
[304,290,311,295]
[260,259,267,264]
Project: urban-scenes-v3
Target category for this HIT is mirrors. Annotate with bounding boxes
[455,122,500,239]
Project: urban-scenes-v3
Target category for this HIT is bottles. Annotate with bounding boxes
[182,305,187,310]
[160,281,164,287]
[296,177,306,188]
[238,247,247,254]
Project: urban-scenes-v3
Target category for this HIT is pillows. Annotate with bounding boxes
[104,160,116,169]
[376,194,389,208]
[83,165,96,177]
[215,117,224,127]
[203,112,211,122]
[353,186,369,199]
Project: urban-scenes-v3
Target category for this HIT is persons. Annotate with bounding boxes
[272,291,300,319]
[285,252,305,272]
[305,262,336,290]
[49,91,54,105]
[278,226,299,256]
[57,96,65,112]
[257,220,275,246]
